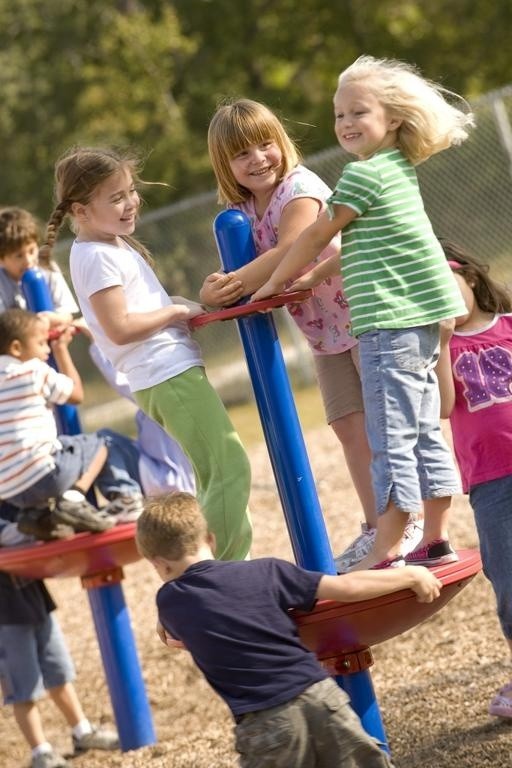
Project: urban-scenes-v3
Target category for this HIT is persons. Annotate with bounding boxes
[35,147,254,564]
[435,239,511,722]
[134,491,445,768]
[247,51,478,573]
[0,207,77,365]
[0,500,121,768]
[0,308,111,542]
[196,98,428,573]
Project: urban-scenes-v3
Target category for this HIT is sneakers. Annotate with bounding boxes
[488,682,512,716]
[404,536,458,566]
[16,508,75,540]
[334,520,378,573]
[338,555,406,576]
[71,727,120,750]
[53,497,113,533]
[31,750,73,767]
[399,519,424,555]
[97,495,144,524]
